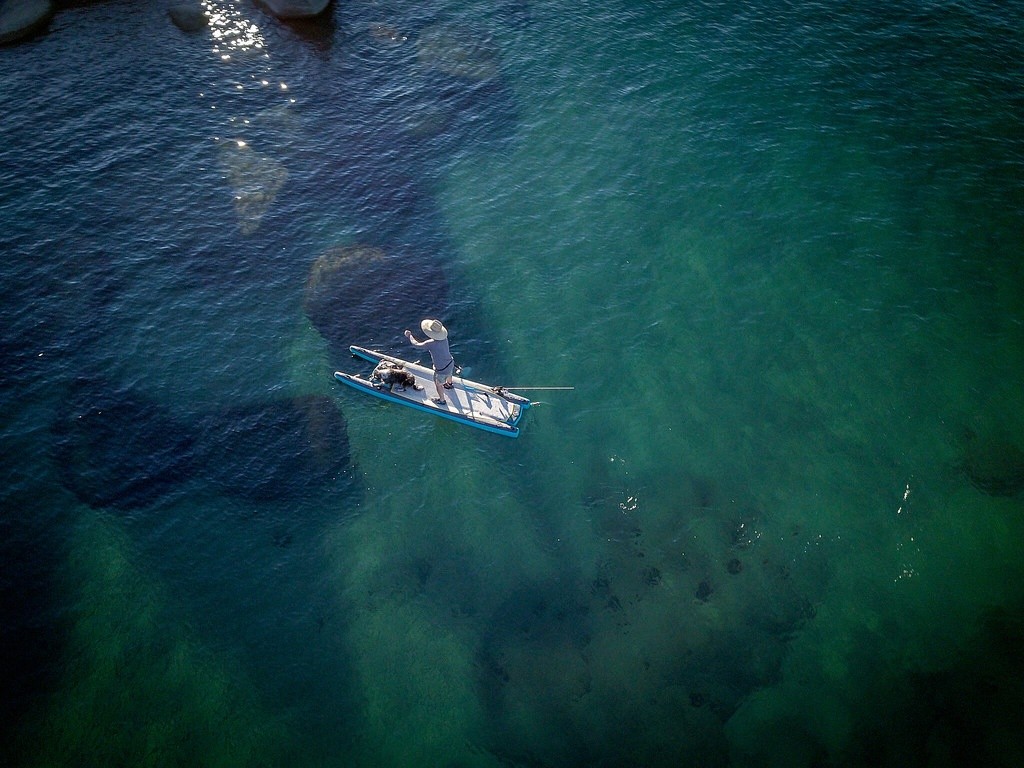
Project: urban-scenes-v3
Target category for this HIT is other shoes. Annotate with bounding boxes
[436,399,446,404]
[443,383,454,389]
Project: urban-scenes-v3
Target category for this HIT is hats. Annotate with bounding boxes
[420,320,447,341]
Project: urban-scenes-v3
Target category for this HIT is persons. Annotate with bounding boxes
[404,319,454,404]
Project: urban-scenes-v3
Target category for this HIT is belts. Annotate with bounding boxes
[438,360,452,371]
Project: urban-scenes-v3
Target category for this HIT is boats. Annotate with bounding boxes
[335,346,535,440]
